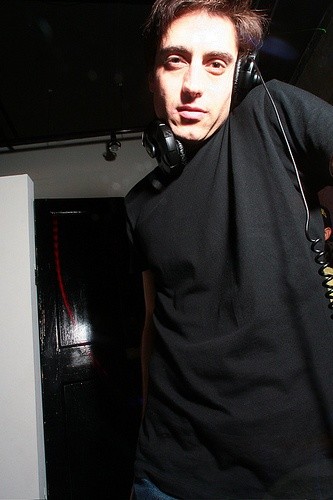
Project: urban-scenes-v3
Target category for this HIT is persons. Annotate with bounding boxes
[98,1,333,500]
[320,204,333,301]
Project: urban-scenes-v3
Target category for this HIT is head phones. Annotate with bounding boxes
[142,50,263,177]
